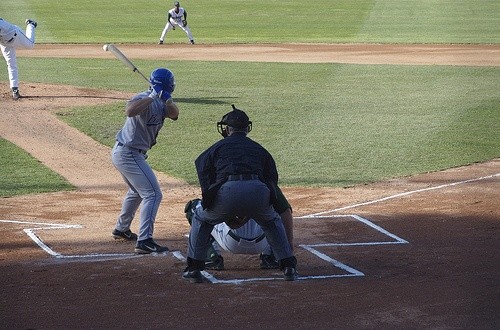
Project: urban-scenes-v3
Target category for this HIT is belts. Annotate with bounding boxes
[228,230,266,243]
[118,143,146,155]
[226,174,258,181]
[6,33,16,43]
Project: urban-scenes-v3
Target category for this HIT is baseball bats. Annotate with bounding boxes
[107,44,173,104]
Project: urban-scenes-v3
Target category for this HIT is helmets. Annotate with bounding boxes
[150,68,175,94]
[217,104,252,136]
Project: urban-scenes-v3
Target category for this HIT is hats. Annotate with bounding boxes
[174,2,179,6]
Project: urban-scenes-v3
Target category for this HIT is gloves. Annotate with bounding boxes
[149,86,171,103]
[148,84,164,101]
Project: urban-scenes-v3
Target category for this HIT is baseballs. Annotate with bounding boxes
[103,44,110,51]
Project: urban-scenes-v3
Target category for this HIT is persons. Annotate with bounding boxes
[159,2,196,45]
[112,68,179,255]
[181,104,300,282]
[0,17,37,99]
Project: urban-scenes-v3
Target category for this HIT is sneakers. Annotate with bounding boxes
[111,228,137,241]
[25,17,37,26]
[204,255,224,269]
[134,238,169,253]
[282,267,299,280]
[259,253,278,269]
[181,269,202,283]
[12,90,21,98]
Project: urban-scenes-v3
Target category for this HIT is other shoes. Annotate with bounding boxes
[159,40,163,44]
[189,39,194,44]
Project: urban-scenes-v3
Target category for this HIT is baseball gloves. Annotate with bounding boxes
[181,20,187,27]
